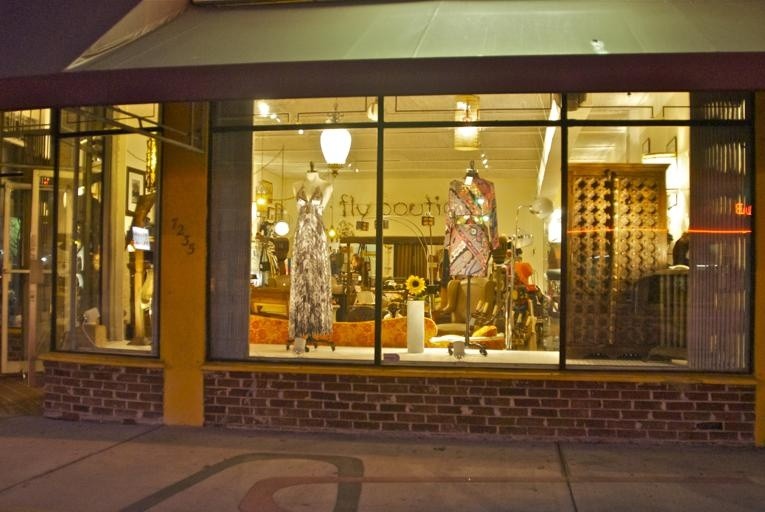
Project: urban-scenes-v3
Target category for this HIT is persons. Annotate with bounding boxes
[673,231,691,268]
[439,249,453,308]
[442,159,501,278]
[666,234,675,265]
[287,161,336,338]
[507,249,550,317]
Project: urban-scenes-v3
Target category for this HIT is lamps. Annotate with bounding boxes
[252,97,561,349]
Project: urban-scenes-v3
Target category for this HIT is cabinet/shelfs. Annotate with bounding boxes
[564,160,670,361]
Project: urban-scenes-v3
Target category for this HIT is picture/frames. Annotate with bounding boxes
[125,166,146,215]
